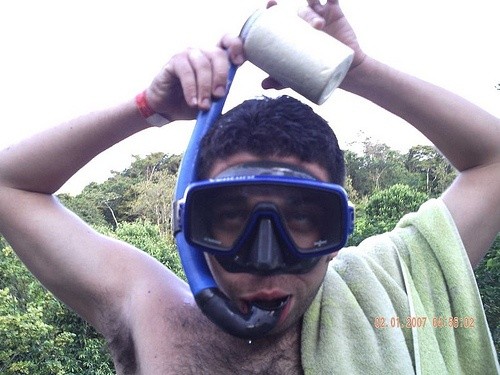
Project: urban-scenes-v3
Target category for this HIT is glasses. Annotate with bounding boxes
[172,36,354,341]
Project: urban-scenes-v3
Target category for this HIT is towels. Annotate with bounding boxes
[301,195,500,375]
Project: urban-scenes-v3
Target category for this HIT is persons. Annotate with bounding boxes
[0,0,500,375]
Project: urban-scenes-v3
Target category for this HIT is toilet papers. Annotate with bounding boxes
[239,4,355,106]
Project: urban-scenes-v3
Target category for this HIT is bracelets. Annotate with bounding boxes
[134,91,171,127]
